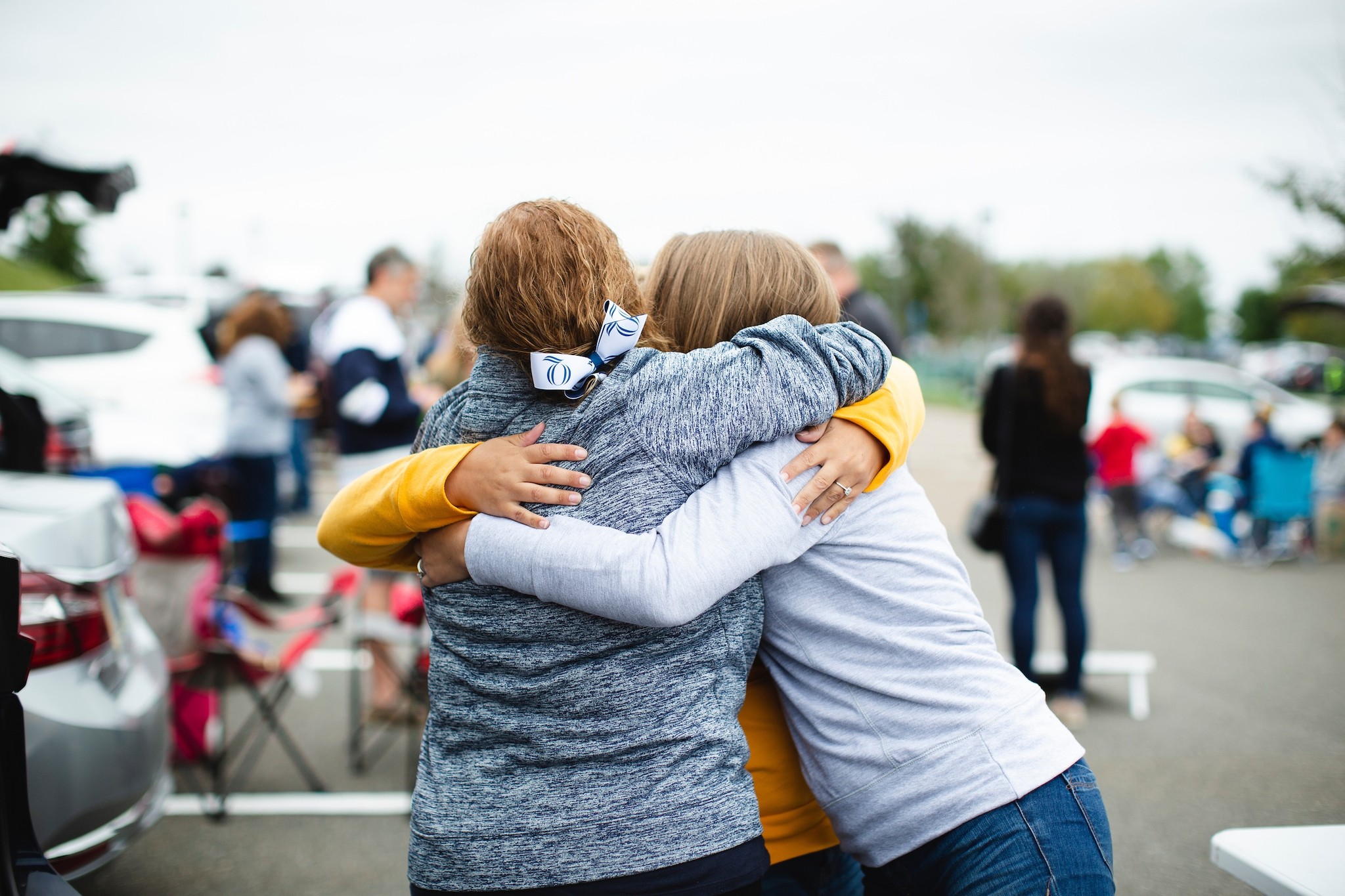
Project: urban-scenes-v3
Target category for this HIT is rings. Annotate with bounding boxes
[835,481,853,495]
[416,559,426,579]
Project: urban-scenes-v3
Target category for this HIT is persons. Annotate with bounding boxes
[314,198,1116,896]
[209,239,454,726]
[1086,380,1345,551]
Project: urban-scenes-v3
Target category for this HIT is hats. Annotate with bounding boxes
[1021,295,1069,333]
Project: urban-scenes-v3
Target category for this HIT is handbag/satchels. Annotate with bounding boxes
[966,498,1003,552]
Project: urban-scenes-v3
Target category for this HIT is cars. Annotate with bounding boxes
[1075,352,1335,460]
[0,466,174,890]
[0,288,246,495]
